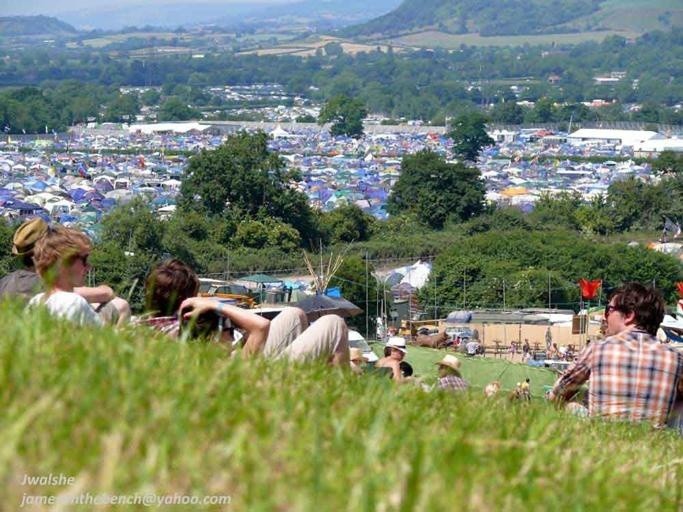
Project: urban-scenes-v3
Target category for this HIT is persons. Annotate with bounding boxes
[260,281,292,301]
[177,296,349,370]
[0,218,132,333]
[546,282,683,434]
[350,308,607,410]
[133,258,202,339]
[23,225,101,331]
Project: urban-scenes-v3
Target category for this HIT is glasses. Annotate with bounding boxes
[604,304,614,318]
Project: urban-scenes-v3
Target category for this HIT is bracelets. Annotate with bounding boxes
[216,302,225,315]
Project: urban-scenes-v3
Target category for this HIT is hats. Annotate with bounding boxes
[385,336,408,354]
[348,347,369,362]
[433,354,461,378]
[11,216,50,256]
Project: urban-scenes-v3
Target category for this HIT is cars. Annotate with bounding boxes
[428,327,477,346]
[345,329,379,366]
